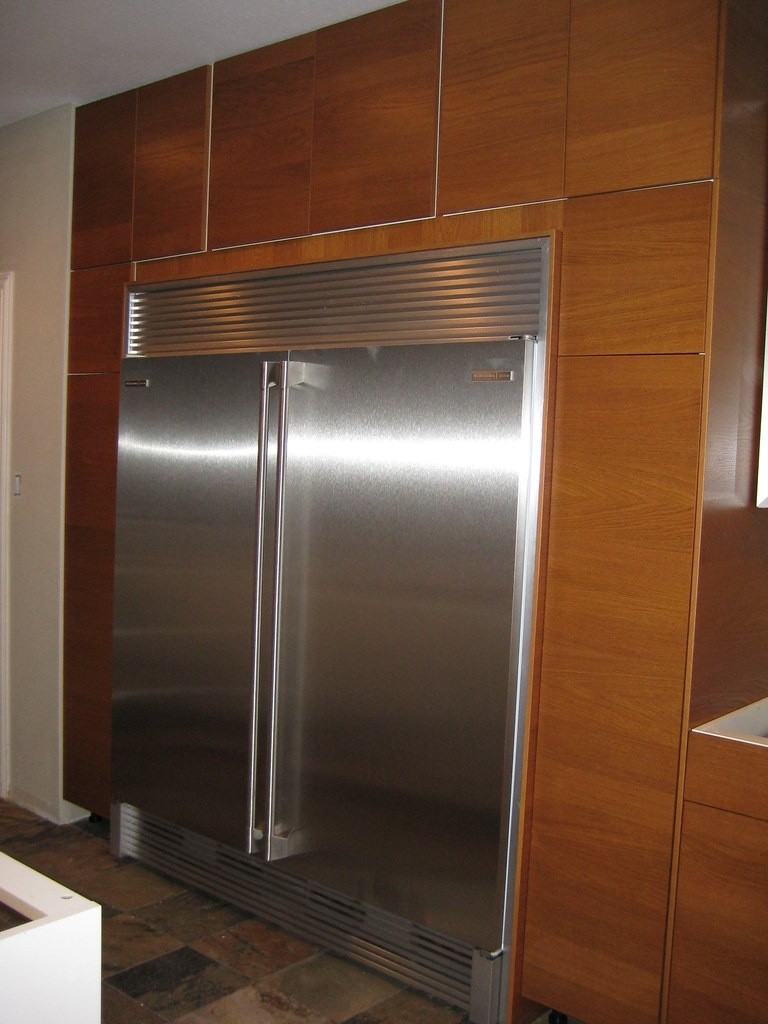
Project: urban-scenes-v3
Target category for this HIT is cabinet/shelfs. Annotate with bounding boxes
[70,0,768,1024]
